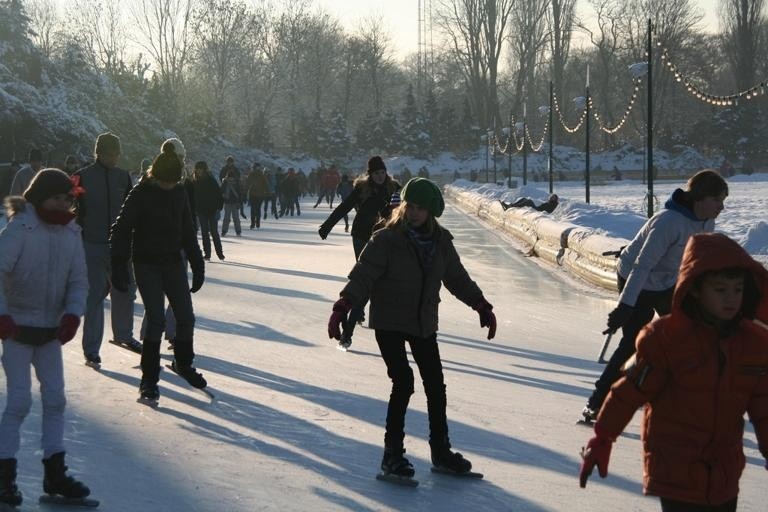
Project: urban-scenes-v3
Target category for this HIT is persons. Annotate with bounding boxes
[318,157,403,325]
[338,168,429,232]
[1,128,270,504]
[454,168,478,183]
[583,168,729,421]
[328,178,496,487]
[501,193,559,213]
[579,231,768,511]
[263,164,340,220]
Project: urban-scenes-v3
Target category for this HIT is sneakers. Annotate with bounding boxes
[263,208,301,219]
[250,221,260,228]
[502,201,512,211]
[582,398,603,421]
[344,225,349,232]
[138,379,162,400]
[113,333,143,355]
[356,309,366,324]
[217,251,225,261]
[82,350,103,364]
[221,229,241,236]
[172,356,208,389]
[202,254,211,261]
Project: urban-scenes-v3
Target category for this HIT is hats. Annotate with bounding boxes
[150,136,189,182]
[94,132,125,157]
[64,154,78,165]
[27,149,44,163]
[386,187,404,211]
[398,175,447,218]
[365,154,388,173]
[23,167,78,204]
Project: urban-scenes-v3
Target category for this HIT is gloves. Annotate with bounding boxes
[57,311,81,347]
[572,423,624,489]
[327,299,350,341]
[0,313,20,344]
[471,295,497,341]
[317,222,331,242]
[602,304,634,336]
[189,259,206,293]
[110,260,134,294]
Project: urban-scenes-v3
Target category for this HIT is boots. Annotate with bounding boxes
[425,432,473,472]
[41,449,92,499]
[376,431,416,476]
[0,457,26,506]
